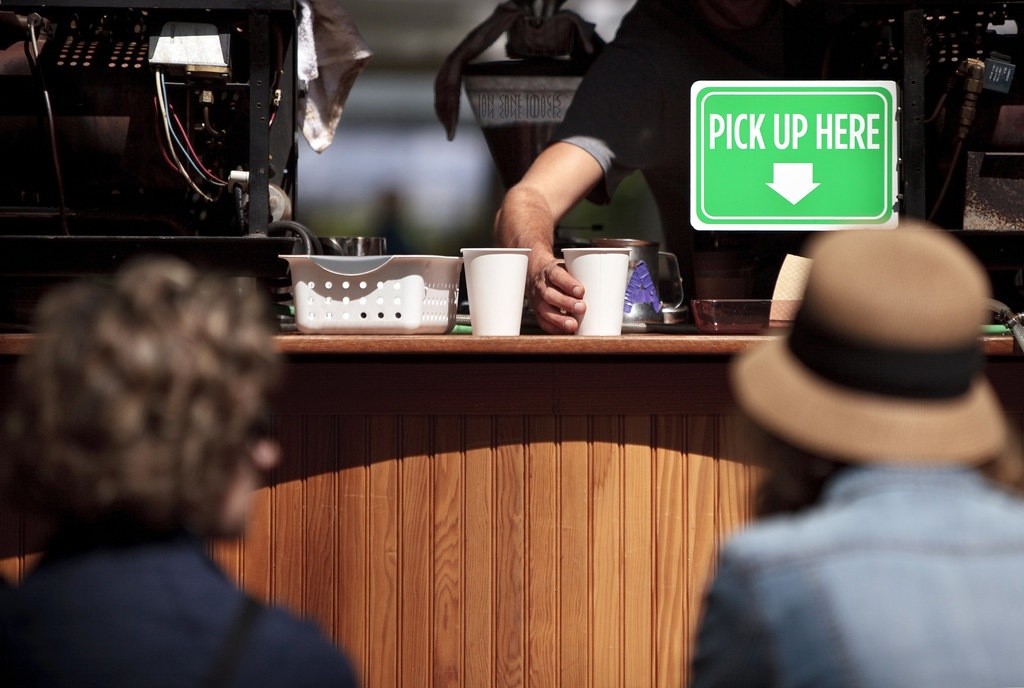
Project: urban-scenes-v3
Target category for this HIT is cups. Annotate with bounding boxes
[562,248,630,338]
[461,248,530,334]
[591,238,659,324]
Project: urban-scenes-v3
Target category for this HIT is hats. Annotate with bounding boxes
[731,221,1007,466]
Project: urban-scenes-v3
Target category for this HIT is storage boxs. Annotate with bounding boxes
[277,252,464,334]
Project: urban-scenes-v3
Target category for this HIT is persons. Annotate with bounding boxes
[1,259,361,688]
[688,221,1024,688]
[494,0,1023,335]
